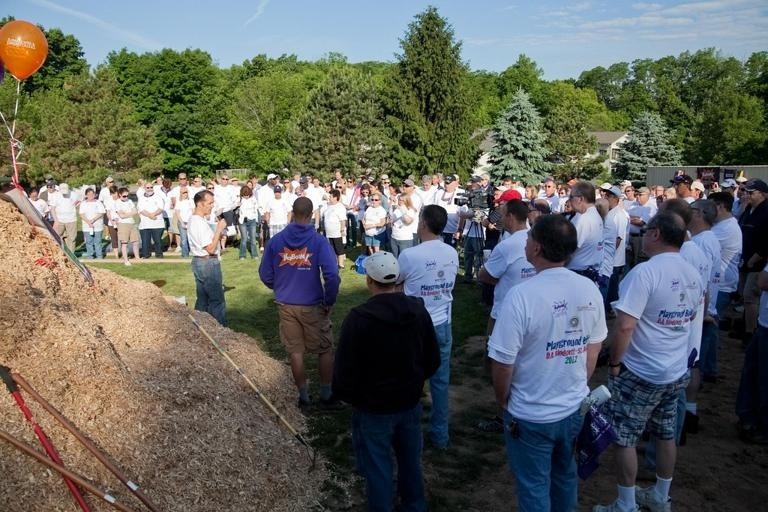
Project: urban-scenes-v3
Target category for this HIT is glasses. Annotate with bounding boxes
[635,193,646,196]
[335,178,389,201]
[639,226,661,237]
[624,190,631,192]
[221,177,229,180]
[206,187,214,189]
[545,185,583,199]
[121,195,128,198]
[179,177,186,180]
[146,186,152,189]
[738,187,746,191]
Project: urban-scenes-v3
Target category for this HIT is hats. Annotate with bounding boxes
[669,175,691,185]
[105,177,113,182]
[492,190,521,203]
[740,181,767,193]
[361,250,399,283]
[266,173,307,192]
[401,174,455,186]
[632,186,649,194]
[45,174,68,195]
[602,186,620,198]
[718,178,736,188]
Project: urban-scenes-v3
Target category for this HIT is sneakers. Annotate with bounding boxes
[317,396,346,410]
[299,396,316,411]
[591,498,641,512]
[634,484,671,512]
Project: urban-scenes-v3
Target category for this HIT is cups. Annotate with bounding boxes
[590,381,611,406]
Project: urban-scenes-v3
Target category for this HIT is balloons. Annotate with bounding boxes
[0,20,49,82]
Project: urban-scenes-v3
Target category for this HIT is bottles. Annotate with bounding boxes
[341,230,347,246]
[112,214,118,231]
[89,223,95,236]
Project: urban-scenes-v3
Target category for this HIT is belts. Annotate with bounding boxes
[629,232,639,237]
[194,255,217,258]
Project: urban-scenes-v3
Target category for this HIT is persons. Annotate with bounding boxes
[330,249,442,512]
[393,205,459,455]
[186,190,233,326]
[478,176,767,482]
[0,168,767,512]
[486,213,609,512]
[591,211,704,512]
[259,197,341,408]
[28,169,489,283]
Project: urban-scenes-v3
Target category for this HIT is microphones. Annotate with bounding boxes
[215,210,229,233]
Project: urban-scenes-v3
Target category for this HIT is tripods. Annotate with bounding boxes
[458,209,486,290]
